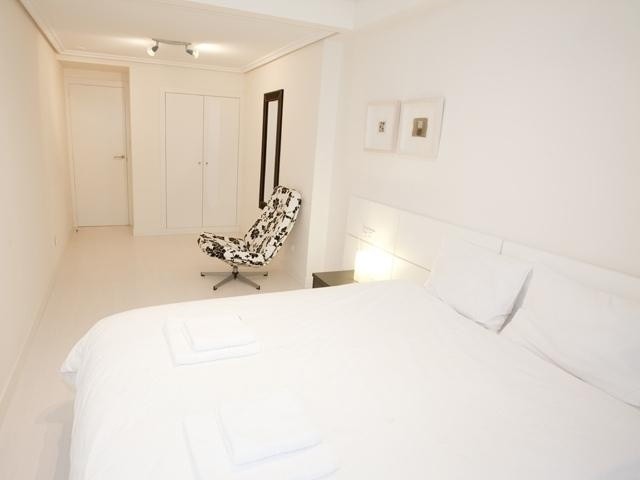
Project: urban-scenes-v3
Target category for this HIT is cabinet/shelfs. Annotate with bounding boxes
[166,93,241,235]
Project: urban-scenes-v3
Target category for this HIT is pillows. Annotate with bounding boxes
[424,235,640,411]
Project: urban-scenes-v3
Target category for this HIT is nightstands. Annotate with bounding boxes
[311,270,355,288]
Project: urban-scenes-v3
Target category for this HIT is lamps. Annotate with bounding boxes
[147,38,198,58]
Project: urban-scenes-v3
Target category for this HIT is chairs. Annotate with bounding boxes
[198,186,303,291]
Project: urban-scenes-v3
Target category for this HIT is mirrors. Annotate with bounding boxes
[258,89,284,208]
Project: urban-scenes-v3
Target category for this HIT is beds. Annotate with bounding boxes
[78,276,638,480]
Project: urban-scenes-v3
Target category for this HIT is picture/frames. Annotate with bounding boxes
[362,98,445,158]
